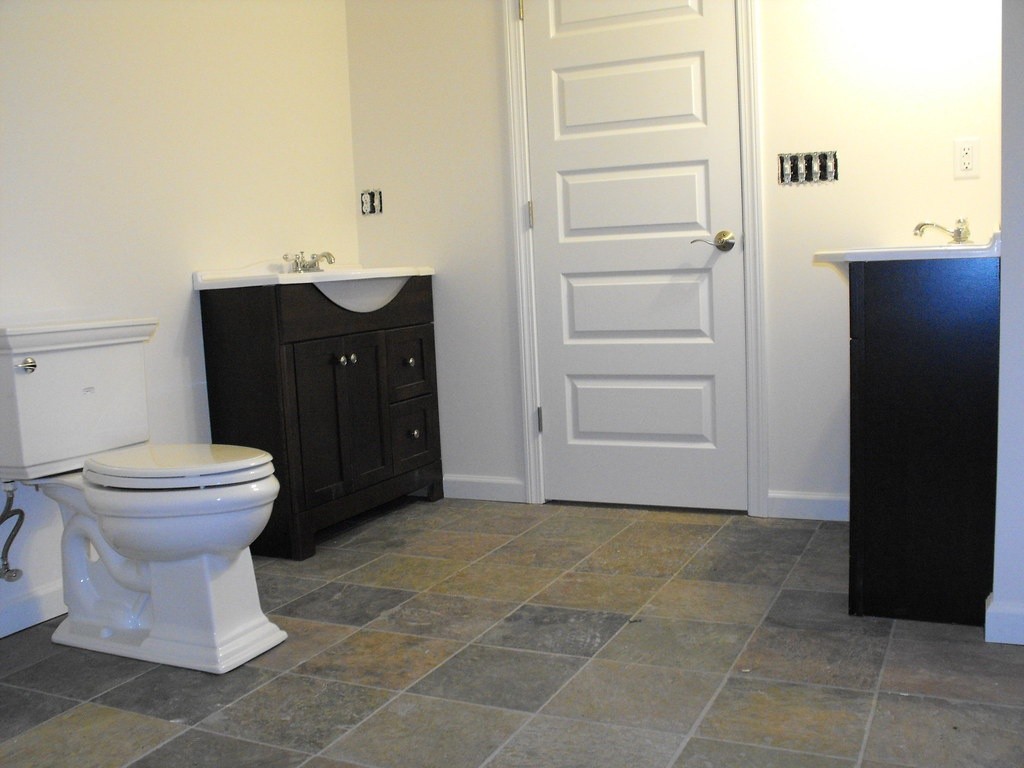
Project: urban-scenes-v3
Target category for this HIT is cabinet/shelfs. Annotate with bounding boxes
[192,265,444,561]
[813,232,1000,623]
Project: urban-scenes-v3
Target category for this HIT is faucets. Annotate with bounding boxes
[305,251,336,270]
[912,216,971,243]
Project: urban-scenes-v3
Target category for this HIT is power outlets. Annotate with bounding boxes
[362,194,371,212]
[953,133,981,179]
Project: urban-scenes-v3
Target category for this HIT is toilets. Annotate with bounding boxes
[0,309,293,676]
